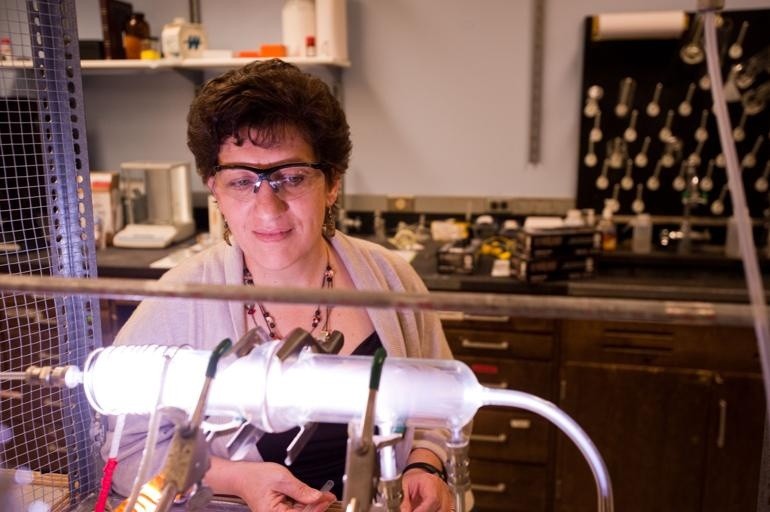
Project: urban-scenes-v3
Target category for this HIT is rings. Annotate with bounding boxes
[449,503,455,512]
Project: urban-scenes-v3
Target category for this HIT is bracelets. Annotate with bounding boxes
[402,461,448,484]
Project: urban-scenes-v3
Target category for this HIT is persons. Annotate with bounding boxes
[96,58,472,512]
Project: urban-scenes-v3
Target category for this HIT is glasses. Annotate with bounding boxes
[211,162,328,193]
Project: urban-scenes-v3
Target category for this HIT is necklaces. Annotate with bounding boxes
[237,248,335,354]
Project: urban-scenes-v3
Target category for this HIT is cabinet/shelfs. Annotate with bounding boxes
[555,320,768,512]
[441,324,554,512]
[1,298,119,468]
[1,57,352,89]
